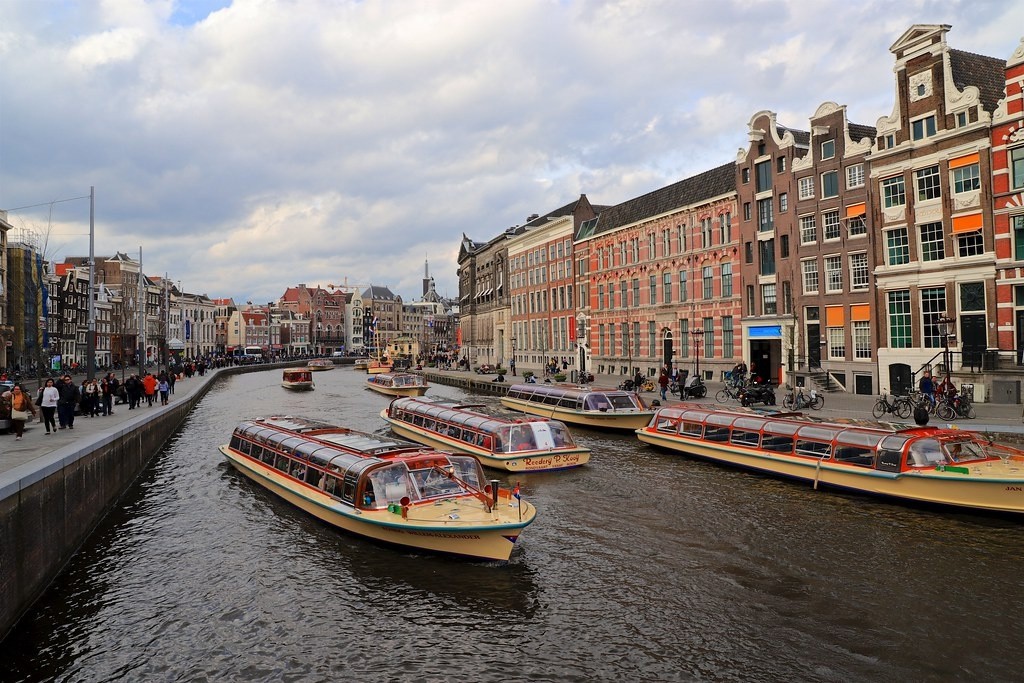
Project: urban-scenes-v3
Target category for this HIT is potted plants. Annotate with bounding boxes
[554,373,567,382]
[497,368,507,375]
[428,363,435,368]
[522,371,534,383]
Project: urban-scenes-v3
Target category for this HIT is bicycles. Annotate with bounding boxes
[4,363,72,383]
[715,376,751,403]
[659,376,685,397]
[933,388,977,421]
[872,388,905,417]
[780,382,826,411]
[896,386,933,419]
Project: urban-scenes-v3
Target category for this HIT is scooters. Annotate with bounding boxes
[618,374,659,394]
[738,378,777,407]
[683,379,708,401]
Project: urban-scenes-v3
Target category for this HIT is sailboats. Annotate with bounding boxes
[364,316,396,374]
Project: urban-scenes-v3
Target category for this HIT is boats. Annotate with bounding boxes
[307,357,336,370]
[499,384,660,431]
[380,394,592,471]
[280,368,316,391]
[354,358,368,371]
[218,416,538,565]
[365,371,432,400]
[632,401,1024,513]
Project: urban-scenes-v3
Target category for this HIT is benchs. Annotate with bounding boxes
[662,425,911,473]
[508,392,582,409]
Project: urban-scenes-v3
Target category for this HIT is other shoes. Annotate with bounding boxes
[128,400,169,410]
[45,433,50,435]
[53,427,57,432]
[102,414,106,416]
[84,414,87,416]
[59,425,67,429]
[95,412,99,416]
[16,436,23,440]
[12,408,29,420]
[108,412,111,415]
[69,426,73,429]
[91,413,94,417]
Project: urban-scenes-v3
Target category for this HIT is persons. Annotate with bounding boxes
[544,357,562,382]
[634,372,642,394]
[913,402,929,427]
[524,374,536,383]
[169,353,276,378]
[920,373,956,415]
[234,431,444,504]
[477,364,488,372]
[732,362,757,386]
[658,364,686,401]
[389,353,468,371]
[397,411,534,451]
[279,353,322,361]
[0,370,176,441]
[498,374,504,382]
[561,359,570,373]
[496,363,501,370]
[510,358,514,372]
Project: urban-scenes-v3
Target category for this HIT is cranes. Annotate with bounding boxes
[327,276,370,292]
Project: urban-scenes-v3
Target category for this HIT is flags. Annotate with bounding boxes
[430,316,433,327]
[370,318,376,335]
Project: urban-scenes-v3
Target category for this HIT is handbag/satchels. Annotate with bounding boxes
[35,387,45,406]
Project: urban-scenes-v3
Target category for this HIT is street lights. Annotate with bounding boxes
[689,328,704,376]
[466,337,472,365]
[509,336,517,376]
[934,313,957,396]
[577,334,586,371]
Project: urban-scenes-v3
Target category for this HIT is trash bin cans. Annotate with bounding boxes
[570,369,578,383]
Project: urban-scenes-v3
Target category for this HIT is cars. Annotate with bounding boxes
[0,381,17,430]
